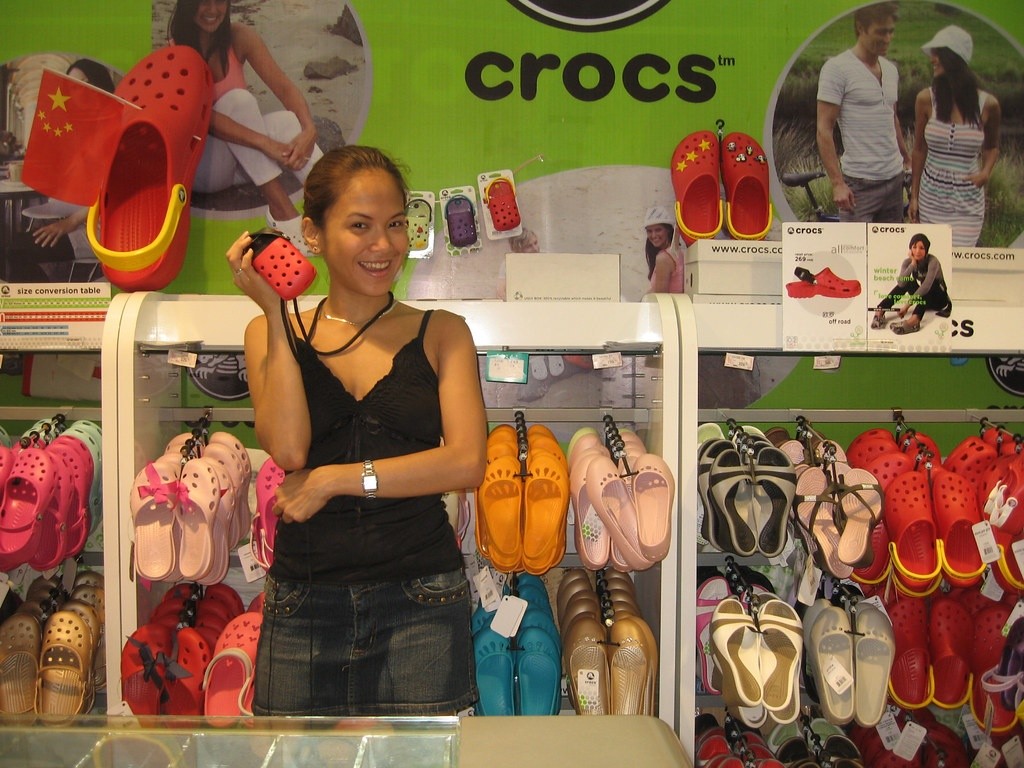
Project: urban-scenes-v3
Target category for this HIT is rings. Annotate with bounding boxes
[236,268,243,274]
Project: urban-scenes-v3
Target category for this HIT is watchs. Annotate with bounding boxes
[361,459,377,499]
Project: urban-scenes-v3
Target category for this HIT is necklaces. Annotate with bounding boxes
[323,299,398,325]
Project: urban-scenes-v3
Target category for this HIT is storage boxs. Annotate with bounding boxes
[951,247,1024,305]
[681,237,783,297]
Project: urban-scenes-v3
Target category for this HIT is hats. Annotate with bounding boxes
[643,205,674,229]
[921,24,973,67]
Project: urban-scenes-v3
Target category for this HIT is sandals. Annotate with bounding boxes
[785,264,863,301]
[239,228,317,301]
[444,195,478,247]
[890,319,921,334]
[265,205,317,259]
[669,132,722,244]
[721,131,774,241]
[871,310,886,329]
[0,419,1023,767]
[404,197,432,251]
[85,45,216,292]
[482,177,522,234]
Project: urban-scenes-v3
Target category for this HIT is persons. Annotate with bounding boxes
[167,0,325,255]
[871,234,951,334]
[7,58,116,283]
[644,204,684,293]
[816,2,909,221]
[226,146,487,715]
[907,25,1001,246]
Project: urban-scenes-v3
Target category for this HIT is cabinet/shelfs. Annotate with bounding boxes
[0,290,1024,768]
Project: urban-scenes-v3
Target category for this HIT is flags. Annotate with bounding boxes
[21,70,124,206]
